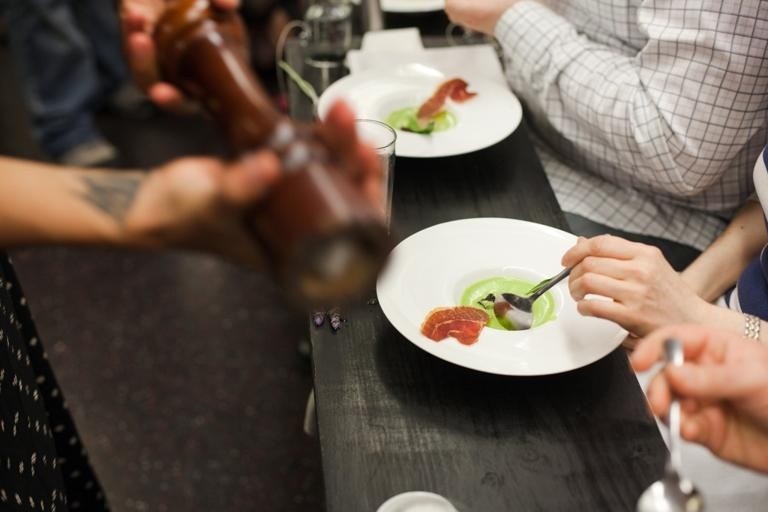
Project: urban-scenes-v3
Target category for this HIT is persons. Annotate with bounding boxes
[560,144,768,511]
[1,0,386,512]
[445,0,768,274]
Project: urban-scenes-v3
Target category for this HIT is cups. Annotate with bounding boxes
[354,122,398,231]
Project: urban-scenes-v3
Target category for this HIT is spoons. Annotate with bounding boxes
[637,338,706,511]
[495,264,577,332]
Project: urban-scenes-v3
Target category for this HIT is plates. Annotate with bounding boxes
[375,218,632,379]
[315,69,521,160]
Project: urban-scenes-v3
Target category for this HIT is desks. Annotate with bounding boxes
[283,34,680,510]
